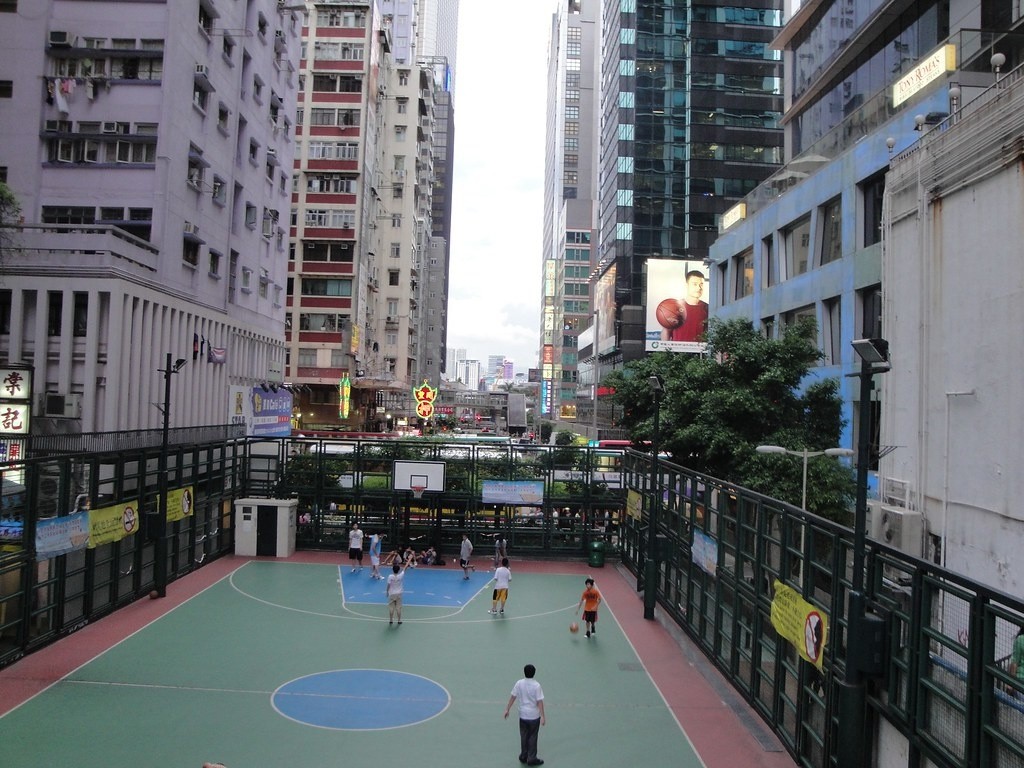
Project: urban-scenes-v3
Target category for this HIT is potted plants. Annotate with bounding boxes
[321,320,326,330]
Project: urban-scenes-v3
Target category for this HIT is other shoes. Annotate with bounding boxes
[519,754,527,762]
[351,568,355,573]
[592,626,596,635]
[584,633,590,638]
[528,760,543,765]
[499,608,504,614]
[376,576,385,580]
[488,608,497,614]
[360,566,363,571]
[373,573,377,577]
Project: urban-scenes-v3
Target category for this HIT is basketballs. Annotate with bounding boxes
[150,590,158,599]
[570,624,579,633]
[655,298,688,330]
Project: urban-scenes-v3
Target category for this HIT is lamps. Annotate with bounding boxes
[298,385,312,393]
[291,385,301,393]
[269,383,277,393]
[294,386,307,393]
[949,87,961,98]
[260,382,269,393]
[991,53,1005,67]
[279,383,294,394]
[914,114,926,125]
[886,137,896,146]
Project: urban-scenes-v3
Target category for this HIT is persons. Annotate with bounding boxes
[575,578,602,639]
[381,545,438,569]
[460,533,476,580]
[495,538,510,570]
[386,555,413,624]
[667,271,708,342]
[504,663,545,767]
[348,522,364,573]
[514,507,609,527]
[488,558,512,615]
[364,531,387,580]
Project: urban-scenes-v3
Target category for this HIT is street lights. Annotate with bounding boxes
[154,352,188,599]
[757,446,857,598]
[644,375,665,621]
[836,337,892,768]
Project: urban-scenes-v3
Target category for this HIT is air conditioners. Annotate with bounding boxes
[355,74,362,80]
[342,42,349,49]
[330,74,337,80]
[184,222,200,237]
[315,42,320,48]
[37,475,60,518]
[386,316,395,322]
[341,244,348,249]
[354,0,420,47]
[330,9,337,15]
[44,393,81,419]
[343,222,349,228]
[276,30,287,41]
[102,121,120,134]
[882,506,923,581]
[396,127,401,132]
[308,243,315,248]
[194,64,210,78]
[290,244,296,249]
[49,30,75,47]
[324,174,340,180]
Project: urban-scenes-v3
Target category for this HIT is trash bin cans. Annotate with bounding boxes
[588,541,605,567]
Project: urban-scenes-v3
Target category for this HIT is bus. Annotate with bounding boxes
[307,410,701,477]
